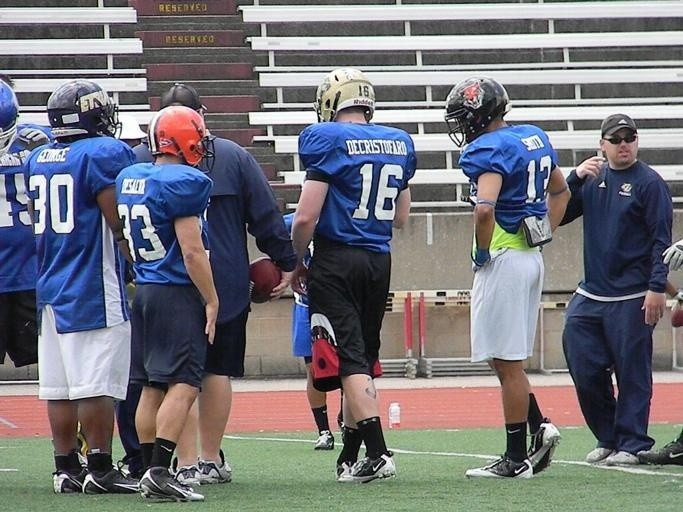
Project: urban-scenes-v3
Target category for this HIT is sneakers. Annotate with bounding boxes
[586,440,682,468]
[465,453,533,480]
[314,410,397,484]
[527,417,560,477]
[51,433,232,503]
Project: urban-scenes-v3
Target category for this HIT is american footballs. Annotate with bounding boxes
[250,259,283,303]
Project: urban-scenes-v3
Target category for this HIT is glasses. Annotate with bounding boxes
[605,134,637,144]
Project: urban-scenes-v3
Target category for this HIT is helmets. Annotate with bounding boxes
[145,106,216,174]
[313,68,376,123]
[46,80,123,143]
[0,79,19,159]
[443,76,513,149]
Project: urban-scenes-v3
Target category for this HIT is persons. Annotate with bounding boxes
[445,77,572,478]
[23,81,141,494]
[113,107,219,502]
[292,67,416,484]
[282,212,346,450]
[636,240,683,465]
[558,113,673,466]
[131,82,298,488]
[1,79,55,367]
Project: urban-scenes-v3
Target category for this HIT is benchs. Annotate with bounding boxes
[0,3,155,160]
[238,1,683,217]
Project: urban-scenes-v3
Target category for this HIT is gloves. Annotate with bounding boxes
[18,128,50,151]
[471,250,491,273]
[662,239,682,272]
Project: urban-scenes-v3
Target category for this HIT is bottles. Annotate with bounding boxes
[387,402,401,429]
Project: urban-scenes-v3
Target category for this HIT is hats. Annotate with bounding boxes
[600,113,637,137]
[159,84,207,110]
